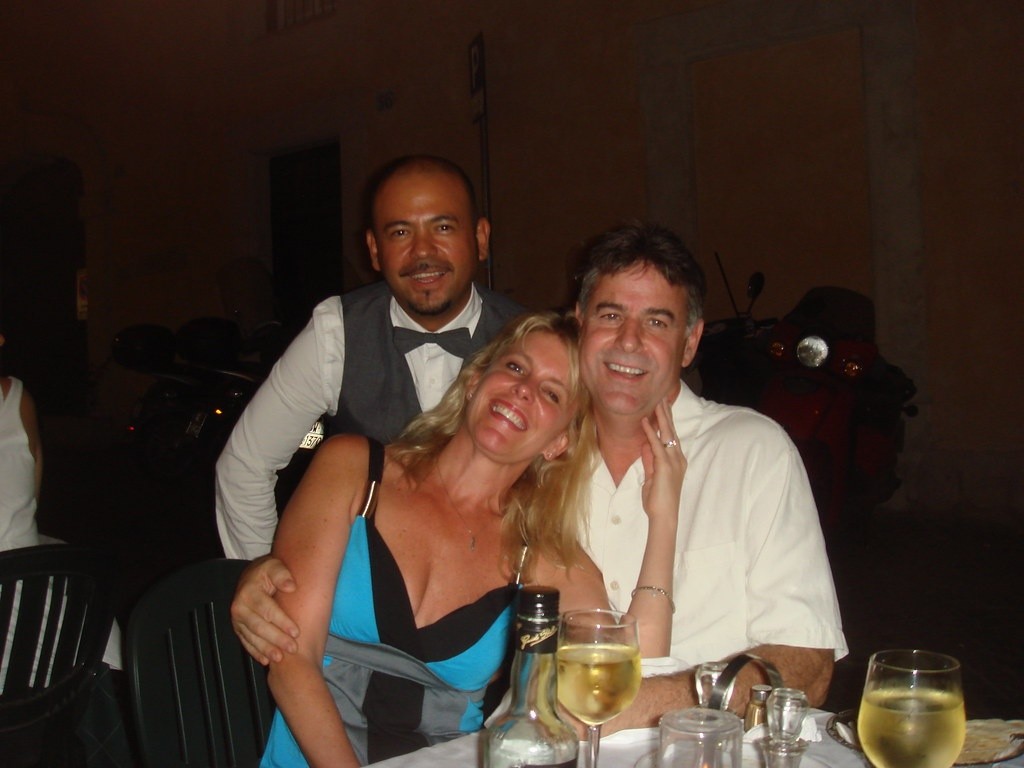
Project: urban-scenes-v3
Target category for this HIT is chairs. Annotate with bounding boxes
[0,545,284,768]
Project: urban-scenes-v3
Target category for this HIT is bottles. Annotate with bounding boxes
[481,586,578,768]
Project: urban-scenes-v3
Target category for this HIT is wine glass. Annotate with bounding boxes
[555,609,643,768]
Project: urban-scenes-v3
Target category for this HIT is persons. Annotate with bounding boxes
[230,222,849,741]
[259,311,687,768]
[0,329,125,680]
[214,156,526,560]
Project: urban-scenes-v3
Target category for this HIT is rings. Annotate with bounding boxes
[663,440,676,448]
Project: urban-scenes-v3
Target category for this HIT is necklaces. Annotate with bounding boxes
[436,460,498,550]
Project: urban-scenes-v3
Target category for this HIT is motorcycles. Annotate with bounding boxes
[109,304,320,500]
[696,252,919,542]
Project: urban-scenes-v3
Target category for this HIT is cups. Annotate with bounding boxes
[659,707,742,768]
[857,650,966,768]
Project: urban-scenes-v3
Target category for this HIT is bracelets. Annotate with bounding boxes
[631,586,676,613]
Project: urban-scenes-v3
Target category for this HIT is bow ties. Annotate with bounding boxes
[393,325,472,358]
